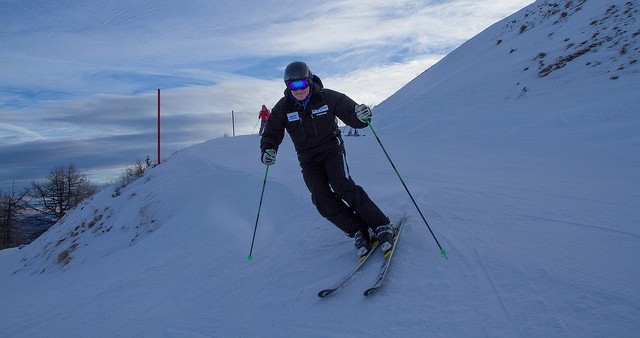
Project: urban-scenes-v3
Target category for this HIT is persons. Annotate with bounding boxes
[348,127,359,135]
[258,105,270,136]
[260,61,398,255]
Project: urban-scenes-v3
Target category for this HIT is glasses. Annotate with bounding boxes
[285,77,310,91]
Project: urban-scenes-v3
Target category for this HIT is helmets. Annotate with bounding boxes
[284,62,313,83]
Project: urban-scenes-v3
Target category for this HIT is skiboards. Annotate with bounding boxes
[318,212,408,297]
[344,134,365,136]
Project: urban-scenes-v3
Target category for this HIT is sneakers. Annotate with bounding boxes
[354,225,375,257]
[376,217,394,250]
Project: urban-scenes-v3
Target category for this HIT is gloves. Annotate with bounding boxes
[355,104,371,123]
[262,149,276,165]
